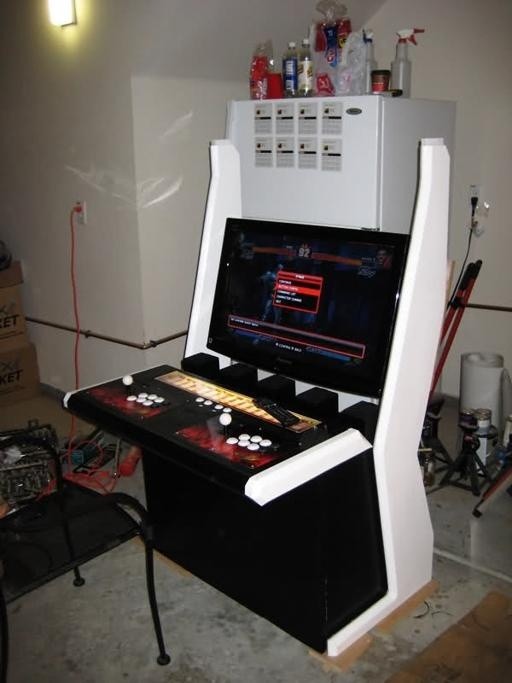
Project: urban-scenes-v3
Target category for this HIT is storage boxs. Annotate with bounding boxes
[0,260,41,406]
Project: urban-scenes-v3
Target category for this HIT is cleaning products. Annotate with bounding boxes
[391,28,424,96]
[282,41,297,94]
[299,39,314,96]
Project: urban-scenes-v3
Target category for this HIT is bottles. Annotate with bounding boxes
[474,407,499,479]
[282,41,298,98]
[297,37,314,98]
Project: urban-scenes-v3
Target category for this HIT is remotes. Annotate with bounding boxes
[252,395,300,427]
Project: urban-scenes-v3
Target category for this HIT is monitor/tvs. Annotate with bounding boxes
[206,217,411,400]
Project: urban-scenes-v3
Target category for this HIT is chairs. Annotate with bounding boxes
[1,424,171,683]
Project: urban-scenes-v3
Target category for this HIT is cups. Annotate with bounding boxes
[370,69,391,94]
